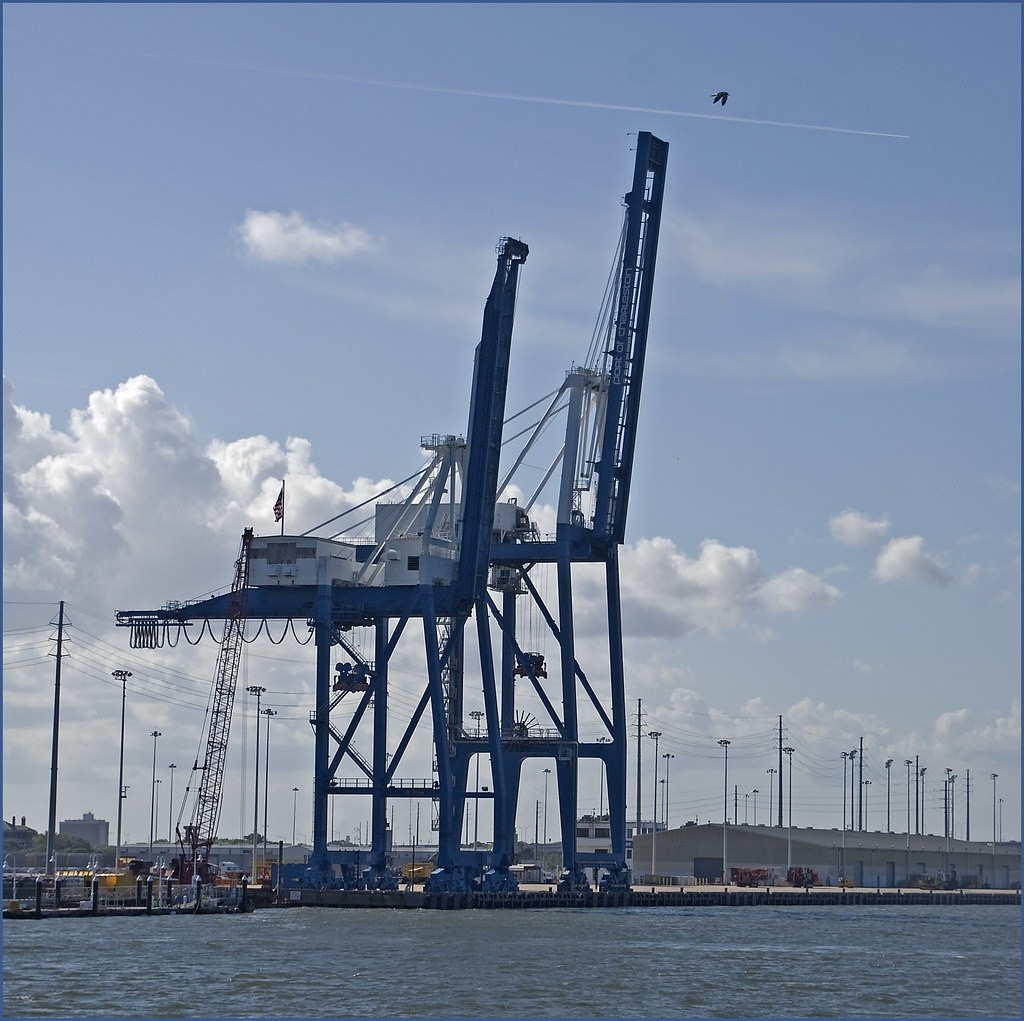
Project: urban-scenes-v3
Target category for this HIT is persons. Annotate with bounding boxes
[242,875,252,883]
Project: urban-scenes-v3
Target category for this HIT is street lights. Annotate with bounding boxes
[752,747,795,868]
[920,767,927,835]
[112,670,133,873]
[292,787,299,845]
[246,686,267,884]
[717,740,731,883]
[840,750,872,877]
[2,848,205,907]
[261,709,278,865]
[648,731,674,875]
[169,764,177,843]
[904,759,913,886]
[885,758,893,833]
[596,736,610,816]
[468,711,484,852]
[542,769,551,868]
[149,731,163,858]
[990,773,1004,890]
[945,767,958,878]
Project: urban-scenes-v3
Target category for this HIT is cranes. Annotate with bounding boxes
[376,128,670,901]
[114,235,532,889]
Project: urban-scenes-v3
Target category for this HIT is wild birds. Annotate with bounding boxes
[710,91,731,107]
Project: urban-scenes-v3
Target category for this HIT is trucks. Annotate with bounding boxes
[221,861,239,875]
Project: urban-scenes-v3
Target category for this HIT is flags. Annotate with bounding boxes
[273,487,283,522]
